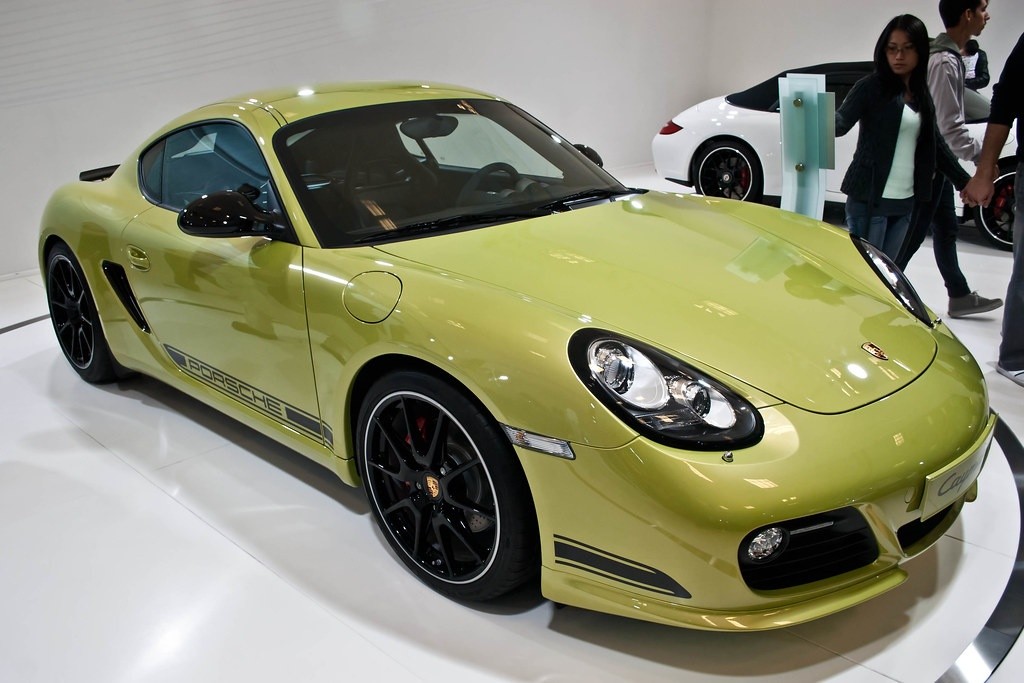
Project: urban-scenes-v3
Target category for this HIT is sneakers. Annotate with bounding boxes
[948,291,1003,318]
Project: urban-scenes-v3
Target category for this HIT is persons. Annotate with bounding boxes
[959,31,1024,386]
[835,14,986,274]
[896,0,1004,317]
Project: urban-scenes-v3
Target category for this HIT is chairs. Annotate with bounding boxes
[200,127,354,250]
[345,120,442,228]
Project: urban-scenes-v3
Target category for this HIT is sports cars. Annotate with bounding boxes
[37,81,997,634]
[650,62,1019,252]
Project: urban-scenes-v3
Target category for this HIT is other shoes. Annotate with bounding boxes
[996,363,1024,386]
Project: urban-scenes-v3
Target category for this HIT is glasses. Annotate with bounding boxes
[884,44,917,56]
